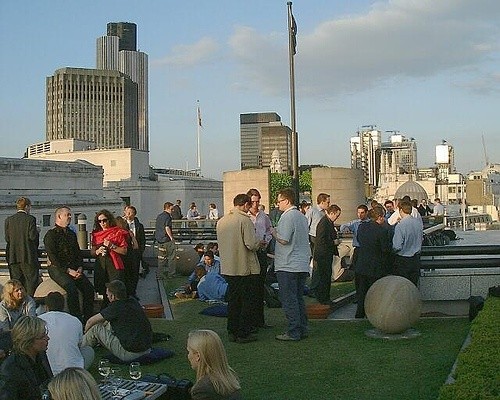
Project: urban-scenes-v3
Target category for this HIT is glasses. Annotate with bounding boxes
[13,287,23,293]
[33,330,49,340]
[276,198,287,204]
[97,218,109,223]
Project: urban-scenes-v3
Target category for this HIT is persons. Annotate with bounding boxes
[152,201,176,279]
[186,241,228,303]
[0,197,153,399]
[340,196,445,318]
[157,330,242,399]
[171,199,218,220]
[216,189,342,342]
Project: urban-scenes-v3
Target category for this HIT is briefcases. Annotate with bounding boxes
[139,372,194,400]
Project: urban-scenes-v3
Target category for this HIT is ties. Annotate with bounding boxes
[128,223,133,230]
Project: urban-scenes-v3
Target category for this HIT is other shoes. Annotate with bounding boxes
[133,294,140,301]
[229,333,258,343]
[321,300,336,305]
[264,324,273,328]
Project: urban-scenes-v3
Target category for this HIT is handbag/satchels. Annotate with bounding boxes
[129,231,139,250]
[108,247,125,270]
[266,297,282,308]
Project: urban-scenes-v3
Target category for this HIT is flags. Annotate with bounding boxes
[290,11,298,58]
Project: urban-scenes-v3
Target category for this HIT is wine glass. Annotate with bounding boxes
[99,359,111,390]
[111,368,122,397]
[130,362,142,391]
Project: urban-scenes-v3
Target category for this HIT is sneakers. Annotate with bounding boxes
[275,331,308,341]
